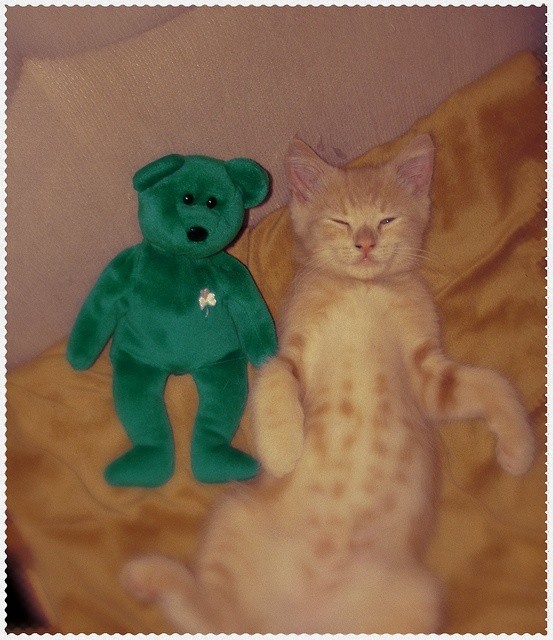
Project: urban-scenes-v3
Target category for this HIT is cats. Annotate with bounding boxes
[121,133,537,634]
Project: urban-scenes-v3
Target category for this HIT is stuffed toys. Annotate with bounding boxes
[65,153,279,489]
